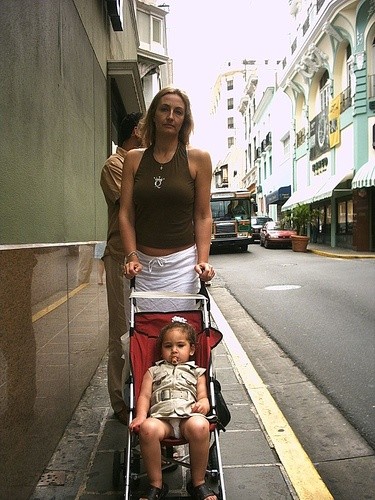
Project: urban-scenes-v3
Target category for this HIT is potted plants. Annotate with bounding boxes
[280,201,321,251]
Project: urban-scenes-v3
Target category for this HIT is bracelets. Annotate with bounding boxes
[128,251,138,257]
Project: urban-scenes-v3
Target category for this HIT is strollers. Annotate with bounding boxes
[114,266,231,500]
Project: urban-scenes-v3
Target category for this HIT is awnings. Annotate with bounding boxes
[267,186,290,204]
[281,172,353,212]
[352,161,375,191]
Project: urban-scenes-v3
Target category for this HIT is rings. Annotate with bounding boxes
[124,272,127,275]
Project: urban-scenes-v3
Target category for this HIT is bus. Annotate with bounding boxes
[209,189,258,251]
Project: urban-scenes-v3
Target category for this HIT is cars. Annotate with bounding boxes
[259,220,297,249]
[252,216,274,240]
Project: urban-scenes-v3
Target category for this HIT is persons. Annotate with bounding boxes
[129,316,217,500]
[100,112,145,424]
[120,88,215,470]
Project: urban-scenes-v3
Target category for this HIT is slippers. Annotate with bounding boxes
[186,479,214,500]
[140,482,168,500]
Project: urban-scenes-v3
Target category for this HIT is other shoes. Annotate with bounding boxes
[115,408,127,425]
